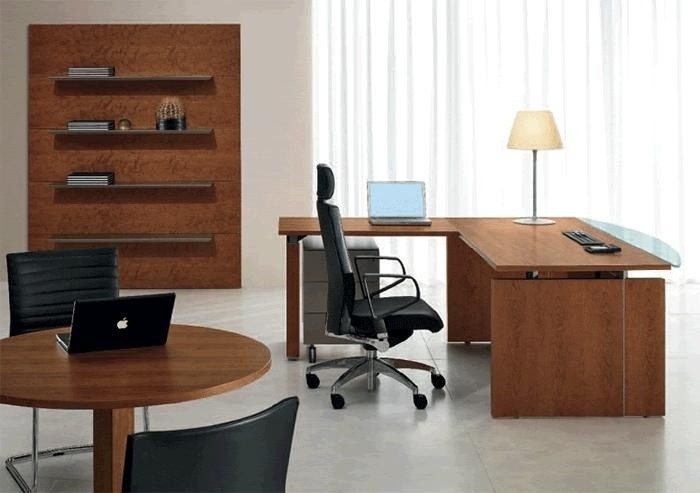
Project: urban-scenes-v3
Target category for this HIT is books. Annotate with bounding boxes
[66,118,114,132]
[65,171,115,187]
[66,65,115,78]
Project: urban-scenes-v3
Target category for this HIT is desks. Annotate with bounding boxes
[278,215,682,418]
[0,321,273,492]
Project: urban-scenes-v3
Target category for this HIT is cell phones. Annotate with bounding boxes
[585,244,621,253]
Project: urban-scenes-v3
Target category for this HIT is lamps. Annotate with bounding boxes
[506,109,564,227]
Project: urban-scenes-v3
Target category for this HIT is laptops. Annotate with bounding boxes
[366,180,433,226]
[56,292,176,355]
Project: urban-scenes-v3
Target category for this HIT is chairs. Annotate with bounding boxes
[4,243,151,493]
[306,161,448,412]
[121,395,300,492]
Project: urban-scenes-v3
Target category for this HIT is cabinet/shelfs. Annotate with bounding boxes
[44,74,218,245]
[302,236,382,365]
